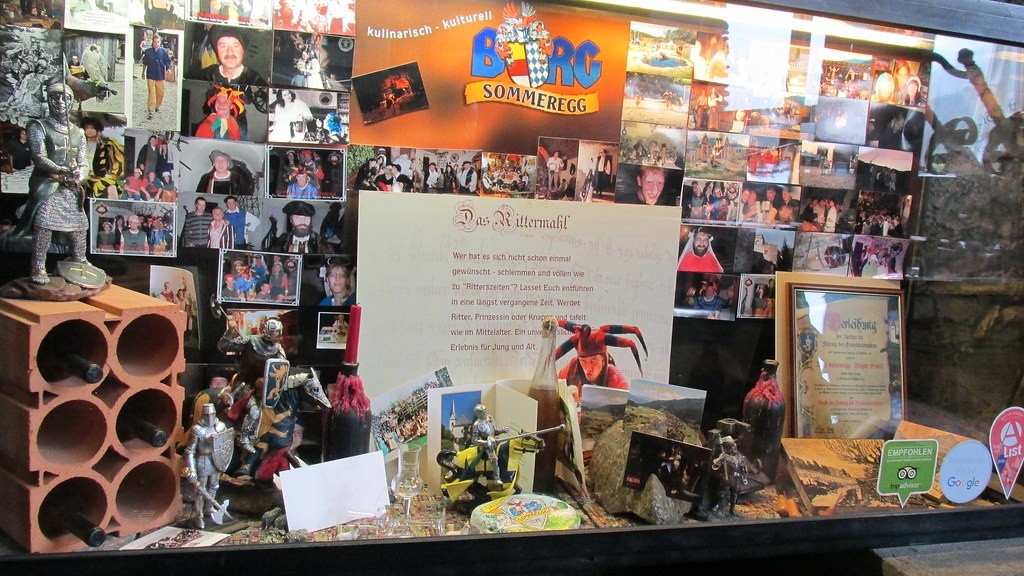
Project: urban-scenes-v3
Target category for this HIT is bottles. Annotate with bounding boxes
[321,360,372,462]
[524,317,560,494]
[741,359,786,485]
[36,331,103,385]
[116,392,167,450]
[40,487,106,549]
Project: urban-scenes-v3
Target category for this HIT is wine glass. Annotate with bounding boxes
[339,444,446,540]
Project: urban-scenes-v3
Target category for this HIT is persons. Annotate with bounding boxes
[25,82,106,285]
[182,404,229,529]
[0,0,927,491]
[217,318,287,454]
[471,404,509,486]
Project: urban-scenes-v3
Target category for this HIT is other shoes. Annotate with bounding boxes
[155,108,162,113]
[147,111,154,117]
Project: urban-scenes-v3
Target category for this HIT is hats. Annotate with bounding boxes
[282,201,316,217]
[223,195,238,202]
[162,171,171,176]
[128,215,139,223]
[80,116,104,132]
[103,222,113,227]
[208,24,249,51]
